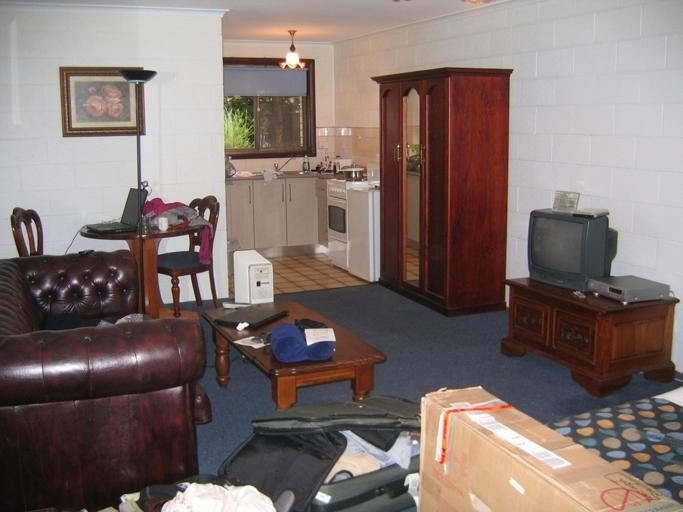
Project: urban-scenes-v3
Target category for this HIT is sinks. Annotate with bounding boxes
[283,170,319,177]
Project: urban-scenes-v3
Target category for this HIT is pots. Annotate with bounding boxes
[342,163,364,181]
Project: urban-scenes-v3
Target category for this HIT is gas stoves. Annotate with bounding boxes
[326,176,378,198]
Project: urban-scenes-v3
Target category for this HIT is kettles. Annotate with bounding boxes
[225,156,236,177]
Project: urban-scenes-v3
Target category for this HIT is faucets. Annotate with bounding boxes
[273,154,295,172]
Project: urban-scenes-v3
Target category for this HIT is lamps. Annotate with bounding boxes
[279,28,306,73]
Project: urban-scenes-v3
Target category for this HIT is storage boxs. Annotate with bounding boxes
[416,384,683,512]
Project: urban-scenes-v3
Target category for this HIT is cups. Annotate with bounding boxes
[158,217,167,232]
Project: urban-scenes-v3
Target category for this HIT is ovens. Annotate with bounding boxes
[326,198,349,273]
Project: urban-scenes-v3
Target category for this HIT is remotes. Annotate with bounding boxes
[572,290,587,299]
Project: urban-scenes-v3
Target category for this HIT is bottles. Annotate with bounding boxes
[138,216,148,234]
[319,156,341,175]
[302,155,311,173]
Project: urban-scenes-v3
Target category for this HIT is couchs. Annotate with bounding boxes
[1,249,207,510]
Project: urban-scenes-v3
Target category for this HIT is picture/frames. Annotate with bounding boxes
[56,62,146,139]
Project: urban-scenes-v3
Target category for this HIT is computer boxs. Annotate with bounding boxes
[233,249,274,305]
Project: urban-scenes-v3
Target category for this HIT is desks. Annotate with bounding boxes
[77,218,206,323]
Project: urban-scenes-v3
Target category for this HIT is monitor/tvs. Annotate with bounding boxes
[527,208,619,293]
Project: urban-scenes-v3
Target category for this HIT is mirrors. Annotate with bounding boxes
[402,88,420,289]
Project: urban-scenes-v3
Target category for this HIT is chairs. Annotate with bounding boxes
[8,207,45,260]
[154,194,221,318]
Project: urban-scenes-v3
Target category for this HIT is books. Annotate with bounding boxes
[215,303,289,330]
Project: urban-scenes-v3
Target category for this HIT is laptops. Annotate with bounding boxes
[216,305,288,329]
[88,187,148,235]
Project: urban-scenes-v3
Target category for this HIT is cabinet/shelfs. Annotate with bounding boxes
[367,67,514,315]
[226,177,254,252]
[499,274,680,397]
[255,175,319,249]
